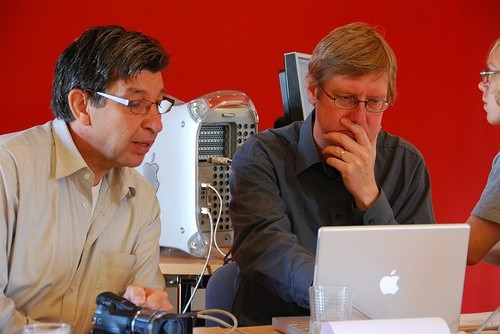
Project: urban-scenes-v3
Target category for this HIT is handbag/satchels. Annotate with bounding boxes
[90,292,194,334]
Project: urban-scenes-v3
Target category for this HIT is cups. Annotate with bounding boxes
[22,323,72,334]
[308,284,354,334]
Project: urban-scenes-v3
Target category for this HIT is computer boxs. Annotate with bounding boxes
[130,90,260,255]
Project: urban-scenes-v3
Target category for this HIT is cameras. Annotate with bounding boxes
[92,292,192,334]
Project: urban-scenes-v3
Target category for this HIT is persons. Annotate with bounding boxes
[462,38,500,268]
[226,23,435,328]
[0,26,173,334]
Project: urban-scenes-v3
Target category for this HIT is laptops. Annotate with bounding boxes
[272,223,470,334]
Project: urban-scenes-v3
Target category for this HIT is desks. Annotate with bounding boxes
[159,247,232,313]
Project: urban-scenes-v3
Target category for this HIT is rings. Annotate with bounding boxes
[339,150,345,157]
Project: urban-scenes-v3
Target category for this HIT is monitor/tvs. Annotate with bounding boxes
[283,50,313,122]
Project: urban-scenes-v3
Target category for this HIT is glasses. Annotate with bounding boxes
[480,70,500,84]
[318,80,393,112]
[83,88,176,114]
